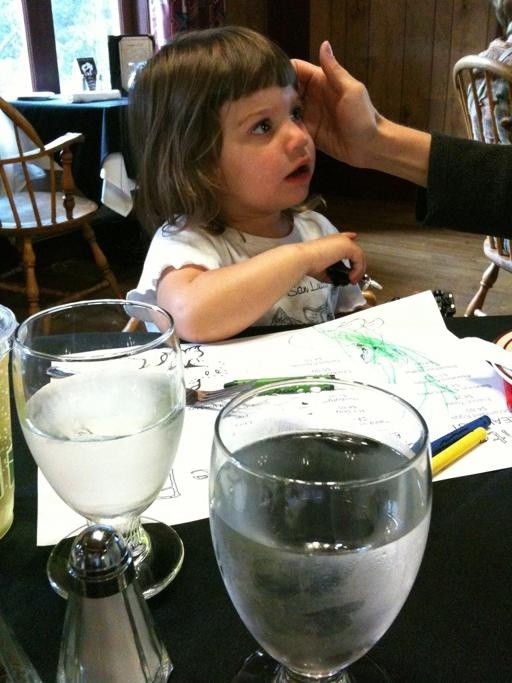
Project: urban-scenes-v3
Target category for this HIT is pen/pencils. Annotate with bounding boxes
[407,415,491,476]
[258,384,333,394]
[225,375,335,386]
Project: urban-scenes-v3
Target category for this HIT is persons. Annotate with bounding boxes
[466,1,511,259]
[276,36,512,241]
[122,24,370,347]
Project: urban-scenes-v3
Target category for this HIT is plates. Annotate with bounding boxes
[17,91,54,101]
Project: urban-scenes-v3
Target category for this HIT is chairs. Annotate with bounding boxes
[452,56,510,317]
[2,99,148,335]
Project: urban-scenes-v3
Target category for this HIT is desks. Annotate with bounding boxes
[9,96,130,170]
[2,317,512,683]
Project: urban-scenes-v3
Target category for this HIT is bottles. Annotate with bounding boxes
[55,522,175,683]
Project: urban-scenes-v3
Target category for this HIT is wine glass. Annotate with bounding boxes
[207,376,434,682]
[11,298,186,600]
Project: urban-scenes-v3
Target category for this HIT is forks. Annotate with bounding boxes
[45,366,254,407]
